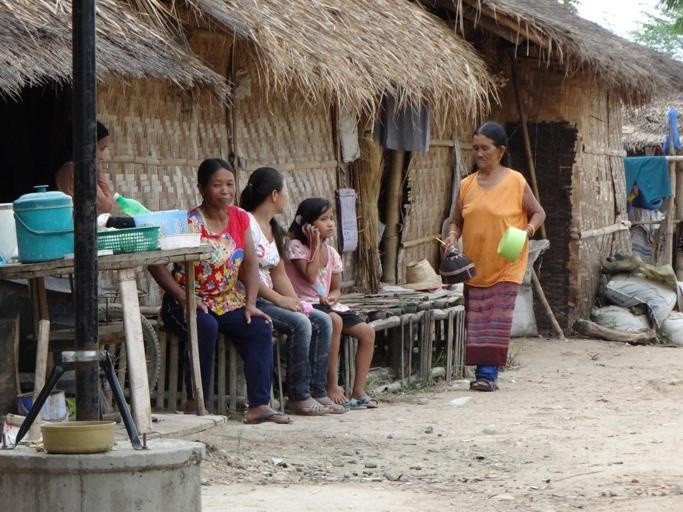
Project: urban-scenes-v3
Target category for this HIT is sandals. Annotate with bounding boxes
[316,397,346,414]
[284,399,334,416]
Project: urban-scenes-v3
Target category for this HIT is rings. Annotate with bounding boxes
[295,305,298,308]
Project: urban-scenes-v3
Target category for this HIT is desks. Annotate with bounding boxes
[0,245,216,436]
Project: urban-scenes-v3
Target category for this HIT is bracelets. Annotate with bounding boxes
[525,222,537,239]
[447,230,460,240]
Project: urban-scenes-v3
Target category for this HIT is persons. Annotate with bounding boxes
[283,196,378,409]
[236,164,351,417]
[437,117,547,392]
[144,156,292,427]
[55,119,122,219]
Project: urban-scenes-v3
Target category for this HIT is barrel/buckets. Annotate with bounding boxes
[13,184,71,262]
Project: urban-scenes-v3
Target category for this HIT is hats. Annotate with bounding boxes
[400,258,443,290]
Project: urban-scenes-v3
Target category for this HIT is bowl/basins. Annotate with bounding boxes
[498,225,528,261]
[39,422,118,454]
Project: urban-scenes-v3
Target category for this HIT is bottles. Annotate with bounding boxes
[112,192,150,217]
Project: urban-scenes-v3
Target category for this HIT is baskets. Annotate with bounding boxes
[97,224,161,255]
[133,209,188,238]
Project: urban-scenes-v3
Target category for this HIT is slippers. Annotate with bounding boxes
[243,412,297,424]
[359,395,379,408]
[342,398,367,410]
[472,379,495,390]
